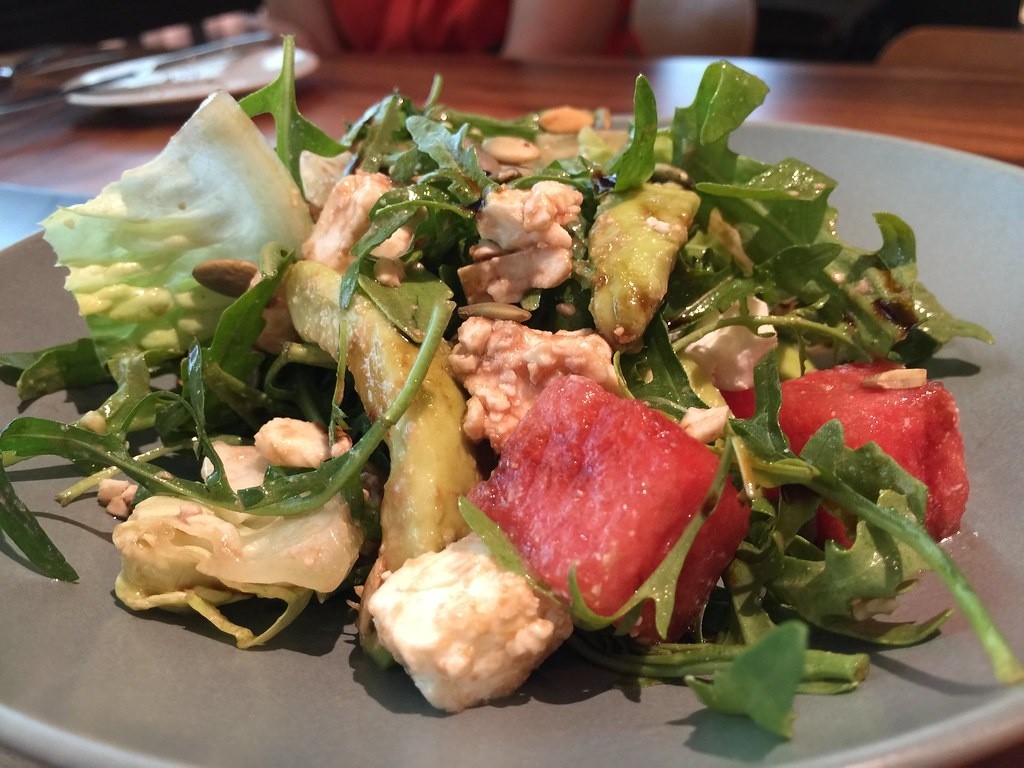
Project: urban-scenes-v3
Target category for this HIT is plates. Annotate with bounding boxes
[0,111,1020,763]
[63,48,313,109]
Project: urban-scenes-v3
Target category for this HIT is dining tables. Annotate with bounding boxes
[1,46,1024,768]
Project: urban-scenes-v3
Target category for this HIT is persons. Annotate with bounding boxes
[135,0,627,62]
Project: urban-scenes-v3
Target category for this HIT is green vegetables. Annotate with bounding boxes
[0,27,1024,740]
